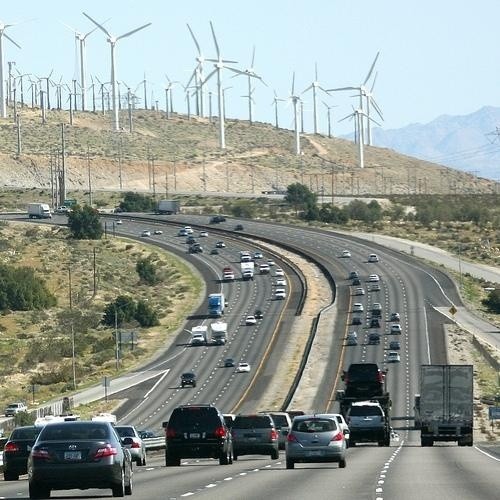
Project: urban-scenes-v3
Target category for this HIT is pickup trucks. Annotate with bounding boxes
[5,402,27,417]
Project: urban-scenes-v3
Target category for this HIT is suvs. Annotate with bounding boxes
[163,404,234,466]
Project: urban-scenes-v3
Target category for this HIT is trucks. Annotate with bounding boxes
[207,293,225,318]
[210,322,229,345]
[417,364,473,447]
[28,203,51,219]
[190,326,209,347]
[154,200,181,215]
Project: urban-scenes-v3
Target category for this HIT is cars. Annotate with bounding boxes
[224,358,235,367]
[181,373,196,388]
[27,421,133,499]
[323,414,351,449]
[223,267,234,281]
[142,226,226,255]
[339,363,392,447]
[113,426,146,466]
[230,413,281,460]
[3,425,45,481]
[341,250,403,362]
[239,250,287,325]
[238,363,250,372]
[282,414,350,469]
[262,412,292,450]
